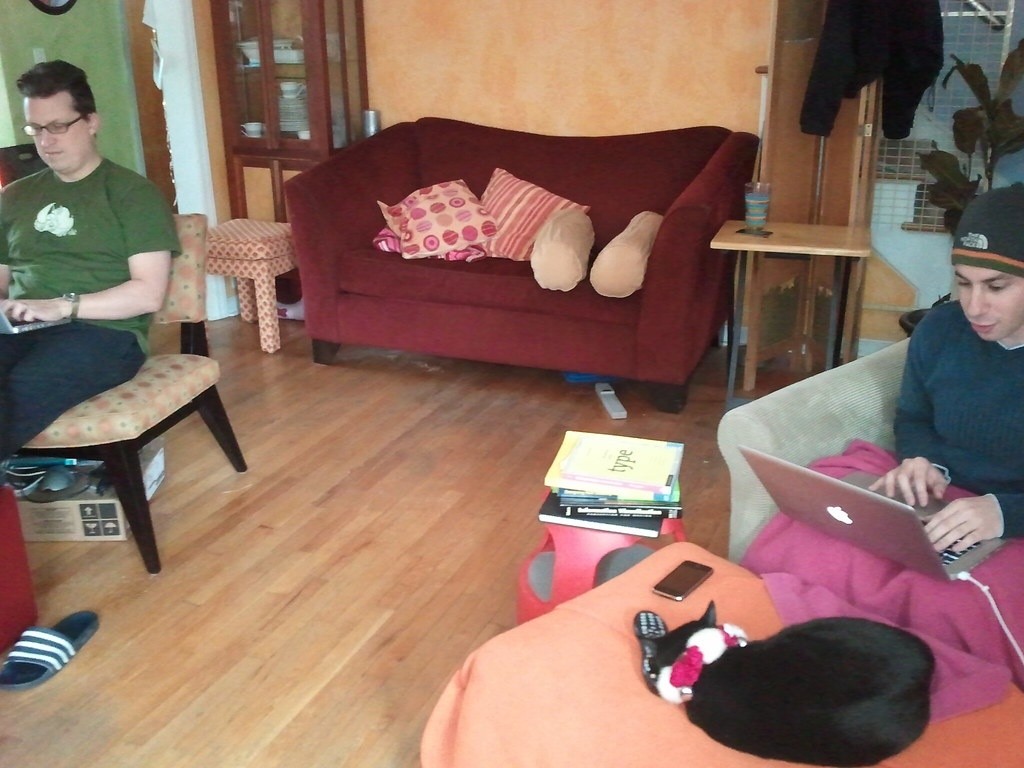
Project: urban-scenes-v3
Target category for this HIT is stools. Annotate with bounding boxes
[426,538,1024,768]
[517,486,686,625]
[202,218,299,354]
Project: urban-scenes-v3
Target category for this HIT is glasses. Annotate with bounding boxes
[21,116,82,136]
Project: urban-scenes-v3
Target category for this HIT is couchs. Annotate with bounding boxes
[716,332,914,569]
[285,117,761,415]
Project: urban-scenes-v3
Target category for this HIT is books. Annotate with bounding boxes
[538,429,686,538]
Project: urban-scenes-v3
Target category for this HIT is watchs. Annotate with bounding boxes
[63,291,80,318]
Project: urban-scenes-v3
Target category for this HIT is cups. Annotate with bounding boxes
[280,82,304,98]
[241,123,262,137]
[297,130,310,139]
[745,182,770,231]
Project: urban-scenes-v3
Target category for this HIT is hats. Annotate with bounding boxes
[951,181,1024,277]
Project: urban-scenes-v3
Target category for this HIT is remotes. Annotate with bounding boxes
[635,610,668,695]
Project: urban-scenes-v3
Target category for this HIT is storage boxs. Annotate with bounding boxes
[17,444,166,544]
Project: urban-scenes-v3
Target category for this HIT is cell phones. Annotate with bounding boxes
[652,561,713,601]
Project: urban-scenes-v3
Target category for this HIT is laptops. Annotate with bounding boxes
[736,444,1007,581]
[0,309,72,335]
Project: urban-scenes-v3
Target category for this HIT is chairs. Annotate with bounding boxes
[12,213,247,574]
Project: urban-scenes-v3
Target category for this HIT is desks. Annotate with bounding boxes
[709,221,869,412]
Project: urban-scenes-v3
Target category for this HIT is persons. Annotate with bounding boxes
[868,182,1024,555]
[0,59,183,465]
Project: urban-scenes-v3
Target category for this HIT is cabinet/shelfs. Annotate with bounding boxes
[207,0,367,321]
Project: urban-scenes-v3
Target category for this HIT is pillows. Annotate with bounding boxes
[376,179,499,260]
[482,166,589,261]
[529,207,595,291]
[589,211,666,298]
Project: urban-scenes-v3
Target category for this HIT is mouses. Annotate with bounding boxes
[39,469,70,492]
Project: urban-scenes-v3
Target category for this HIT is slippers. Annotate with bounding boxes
[0,611,100,691]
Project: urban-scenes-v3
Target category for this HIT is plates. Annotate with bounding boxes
[278,95,309,134]
